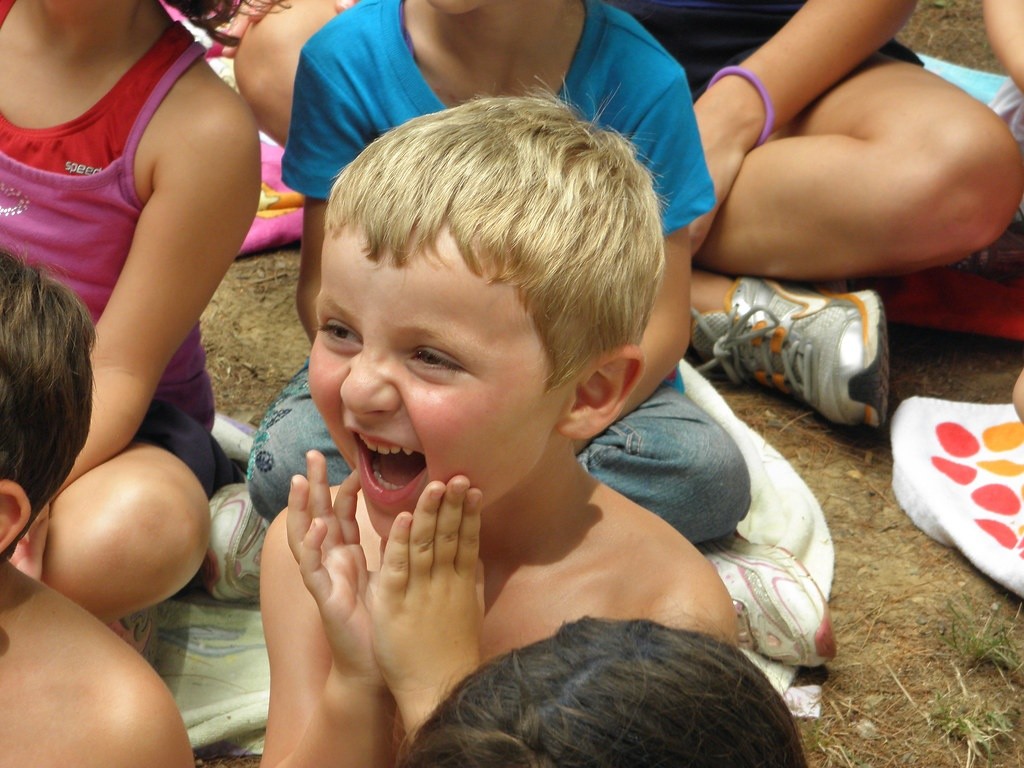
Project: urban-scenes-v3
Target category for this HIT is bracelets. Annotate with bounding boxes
[711,65,774,149]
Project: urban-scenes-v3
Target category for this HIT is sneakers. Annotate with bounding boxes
[690,276,890,431]
[207,483,270,604]
[690,529,836,668]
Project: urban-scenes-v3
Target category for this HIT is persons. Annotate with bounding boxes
[1,245,197,768]
[0,1,1022,768]
